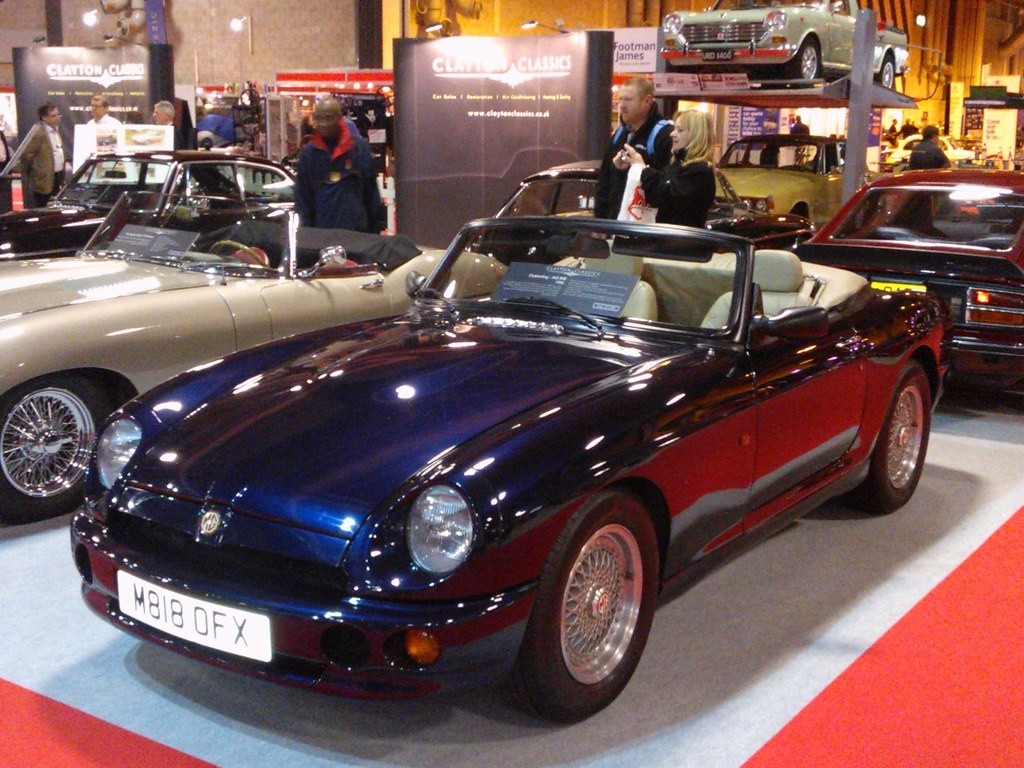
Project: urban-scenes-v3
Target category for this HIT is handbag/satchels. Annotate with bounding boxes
[615,163,659,239]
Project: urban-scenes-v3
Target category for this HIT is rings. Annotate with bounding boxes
[621,157,626,162]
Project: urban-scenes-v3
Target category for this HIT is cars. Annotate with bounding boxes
[887,134,974,163]
[711,134,878,233]
[493,156,811,249]
[73,218,942,724]
[791,167,1024,386]
[0,150,298,261]
[661,0,909,91]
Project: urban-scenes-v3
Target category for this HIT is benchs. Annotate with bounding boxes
[132,191,228,216]
[641,258,737,329]
[220,241,369,270]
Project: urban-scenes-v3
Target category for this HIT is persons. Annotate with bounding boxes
[293,96,387,268]
[790,115,808,134]
[895,118,918,140]
[909,125,952,170]
[623,111,718,227]
[887,119,897,134]
[593,75,675,224]
[85,94,122,125]
[152,100,186,150]
[0,102,72,206]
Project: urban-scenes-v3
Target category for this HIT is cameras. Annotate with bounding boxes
[620,151,629,163]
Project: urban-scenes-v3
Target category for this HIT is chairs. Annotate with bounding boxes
[700,248,808,329]
[582,238,660,322]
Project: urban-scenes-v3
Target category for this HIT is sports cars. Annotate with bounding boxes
[131,132,162,144]
[0,191,512,523]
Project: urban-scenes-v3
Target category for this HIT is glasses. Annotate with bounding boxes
[50,114,62,120]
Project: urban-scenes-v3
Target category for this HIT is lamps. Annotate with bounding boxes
[231,15,253,54]
[522,20,569,34]
[33,37,47,43]
[422,7,443,32]
[915,14,926,26]
[103,35,143,46]
